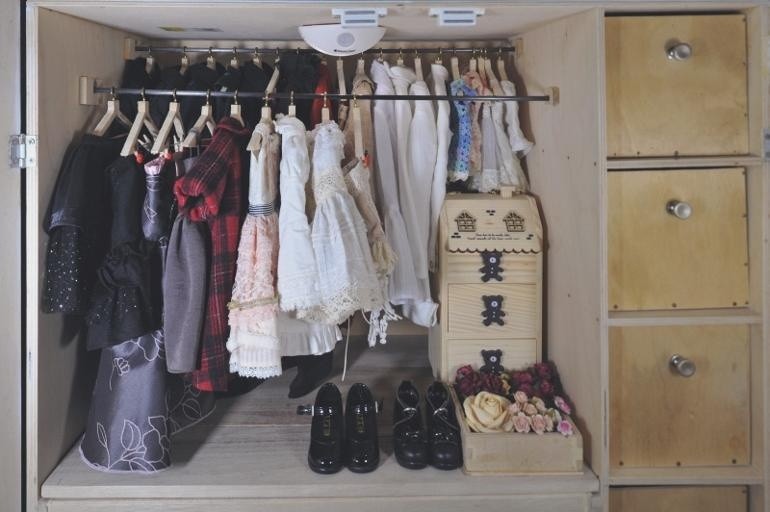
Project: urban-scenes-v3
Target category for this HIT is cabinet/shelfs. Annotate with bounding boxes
[603,14,764,512]
[425,189,545,388]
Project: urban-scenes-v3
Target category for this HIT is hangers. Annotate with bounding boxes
[246,92,276,151]
[182,90,217,150]
[91,84,133,139]
[151,88,186,157]
[120,86,158,158]
[228,88,245,128]
[288,90,298,117]
[352,91,367,159]
[322,90,331,123]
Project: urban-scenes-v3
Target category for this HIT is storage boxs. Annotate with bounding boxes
[451,381,585,475]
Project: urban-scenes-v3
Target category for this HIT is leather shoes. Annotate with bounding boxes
[344,381,385,474]
[296,381,345,475]
[391,379,429,471]
[424,380,462,471]
